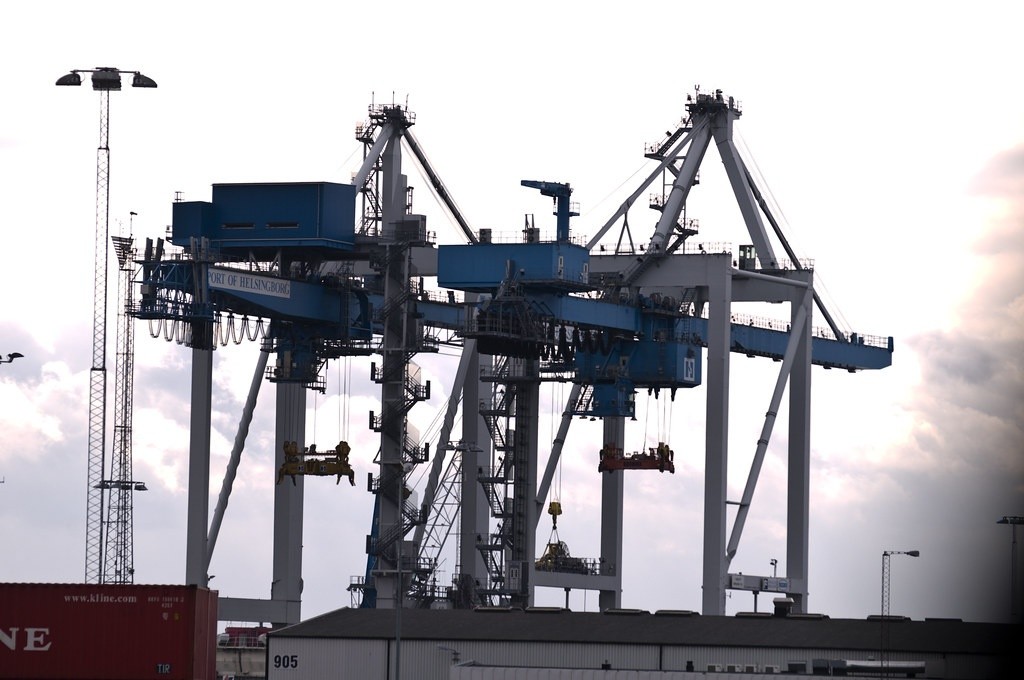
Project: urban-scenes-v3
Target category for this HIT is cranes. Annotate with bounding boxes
[880,551,920,673]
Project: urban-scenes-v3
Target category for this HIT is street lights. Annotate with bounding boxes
[54,67,158,583]
[996,516,1024,622]
